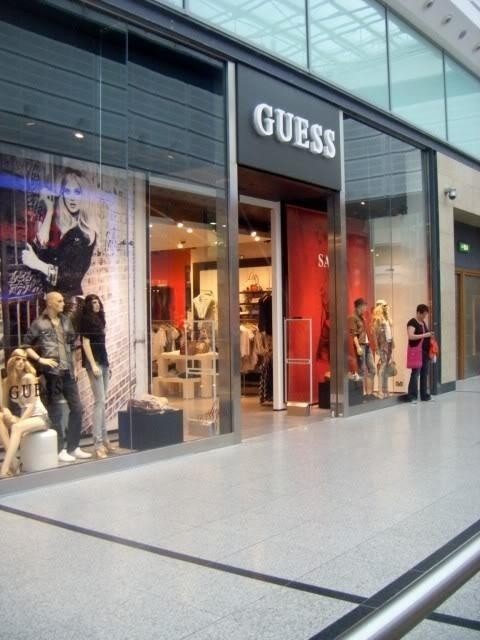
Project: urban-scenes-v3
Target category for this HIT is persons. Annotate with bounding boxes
[21,162,98,345]
[77,293,122,460]
[347,296,379,403]
[1,346,55,479]
[370,299,397,400]
[21,289,93,463]
[404,304,435,404]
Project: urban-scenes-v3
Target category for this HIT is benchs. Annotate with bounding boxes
[153,377,201,400]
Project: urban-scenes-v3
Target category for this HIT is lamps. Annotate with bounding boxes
[444,188,456,199]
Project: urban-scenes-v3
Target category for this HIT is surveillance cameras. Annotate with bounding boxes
[448,191,457,200]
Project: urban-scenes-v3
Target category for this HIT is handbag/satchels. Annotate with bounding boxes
[377,357,398,377]
[242,273,263,291]
[407,346,423,369]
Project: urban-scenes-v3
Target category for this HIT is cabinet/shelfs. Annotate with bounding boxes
[240,290,271,316]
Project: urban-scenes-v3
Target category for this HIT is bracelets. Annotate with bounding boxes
[36,356,42,362]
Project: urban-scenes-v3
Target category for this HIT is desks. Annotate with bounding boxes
[158,352,218,397]
